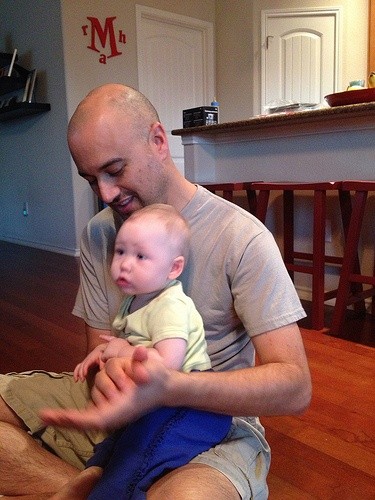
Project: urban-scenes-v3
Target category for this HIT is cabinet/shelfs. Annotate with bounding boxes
[1,52,50,121]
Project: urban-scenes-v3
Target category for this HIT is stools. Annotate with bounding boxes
[250,180,367,330]
[328,180,375,339]
[190,181,264,216]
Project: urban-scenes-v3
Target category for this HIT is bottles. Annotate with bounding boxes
[211,96,222,124]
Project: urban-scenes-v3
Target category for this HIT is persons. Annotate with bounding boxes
[50,204,232,500]
[0,83,314,500]
